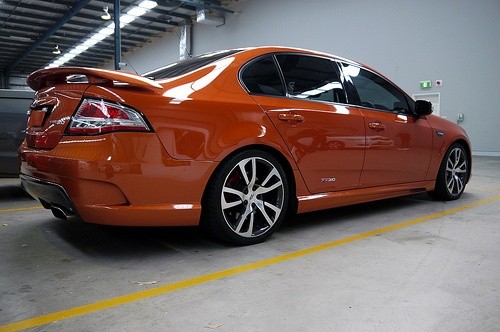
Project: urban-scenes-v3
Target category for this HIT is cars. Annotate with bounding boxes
[19,46,472,244]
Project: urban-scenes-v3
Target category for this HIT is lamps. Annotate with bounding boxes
[53,44,62,55]
[100,3,112,20]
